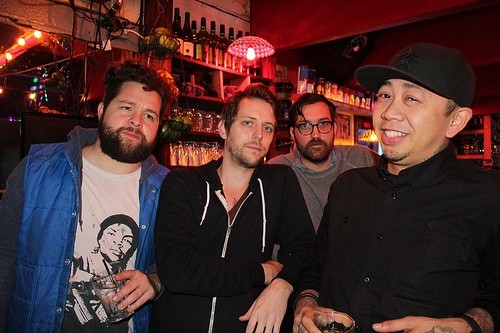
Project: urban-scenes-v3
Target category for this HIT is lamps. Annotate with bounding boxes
[227,36,275,60]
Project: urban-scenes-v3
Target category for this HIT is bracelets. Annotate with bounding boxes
[294,293,319,309]
[458,314,482,333]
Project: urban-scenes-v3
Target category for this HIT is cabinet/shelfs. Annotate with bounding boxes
[157,50,272,171]
[451,102,500,170]
[274,69,383,159]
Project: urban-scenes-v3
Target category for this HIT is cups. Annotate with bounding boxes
[170,105,224,167]
[313,312,355,333]
[90,266,135,323]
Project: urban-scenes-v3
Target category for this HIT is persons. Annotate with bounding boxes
[0,60,171,333]
[152,82,319,333]
[292,41,500,333]
[266,93,382,233]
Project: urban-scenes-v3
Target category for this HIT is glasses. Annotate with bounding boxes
[292,120,334,135]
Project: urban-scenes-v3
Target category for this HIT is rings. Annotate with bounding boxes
[298,322,306,333]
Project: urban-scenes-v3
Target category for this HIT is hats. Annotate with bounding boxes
[355,44,474,108]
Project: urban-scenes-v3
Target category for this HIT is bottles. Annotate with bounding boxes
[461,135,500,156]
[171,7,260,76]
[298,65,372,111]
